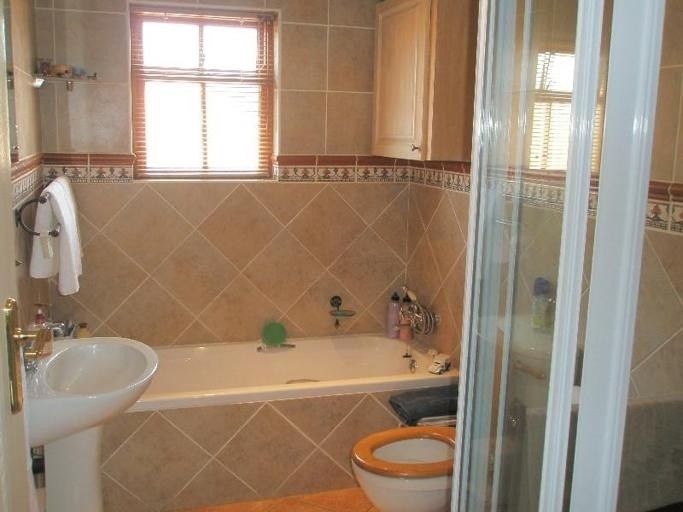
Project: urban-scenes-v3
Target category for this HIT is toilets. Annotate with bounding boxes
[351,313,586,512]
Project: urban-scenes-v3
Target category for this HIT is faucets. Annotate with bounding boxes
[24,321,68,372]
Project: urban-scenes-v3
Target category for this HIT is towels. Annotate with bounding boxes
[29,176,84,297]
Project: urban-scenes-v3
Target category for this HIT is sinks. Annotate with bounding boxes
[20,335,159,447]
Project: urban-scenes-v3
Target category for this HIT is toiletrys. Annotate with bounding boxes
[385,293,400,337]
[28,302,55,356]
[400,293,413,341]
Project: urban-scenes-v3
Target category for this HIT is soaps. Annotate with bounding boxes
[329,309,355,316]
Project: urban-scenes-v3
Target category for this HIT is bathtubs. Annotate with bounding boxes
[118,331,460,415]
